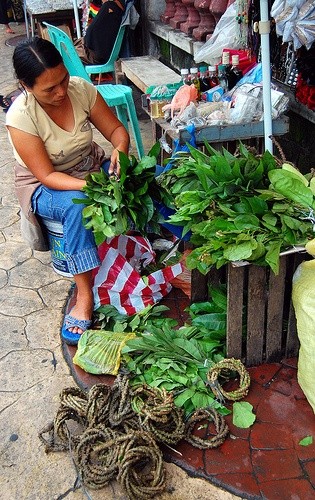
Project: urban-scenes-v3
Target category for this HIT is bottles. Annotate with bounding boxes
[143,51,245,120]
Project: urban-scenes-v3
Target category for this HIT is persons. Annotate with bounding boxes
[0,0,15,34]
[4,37,192,347]
[0,0,126,113]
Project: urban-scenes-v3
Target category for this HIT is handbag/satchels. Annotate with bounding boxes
[91,234,182,316]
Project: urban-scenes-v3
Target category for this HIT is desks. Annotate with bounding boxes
[24,0,81,40]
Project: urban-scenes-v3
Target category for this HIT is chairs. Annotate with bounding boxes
[84,1,133,85]
[43,22,145,163]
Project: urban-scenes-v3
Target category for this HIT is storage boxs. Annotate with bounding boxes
[189,237,307,383]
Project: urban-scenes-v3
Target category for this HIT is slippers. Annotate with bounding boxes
[0,89,23,108]
[61,304,93,346]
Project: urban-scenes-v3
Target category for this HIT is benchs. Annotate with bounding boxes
[114,55,182,94]
